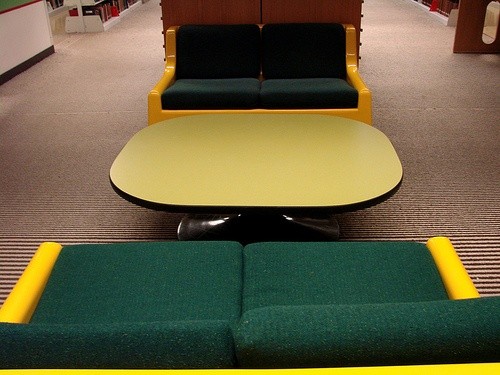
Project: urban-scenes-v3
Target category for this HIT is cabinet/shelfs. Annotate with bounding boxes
[80,0,145,35]
[47,0,64,15]
[412,0,471,22]
[64,6,81,33]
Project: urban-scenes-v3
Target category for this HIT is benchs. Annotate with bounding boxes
[147,21,371,131]
[2,234,500,375]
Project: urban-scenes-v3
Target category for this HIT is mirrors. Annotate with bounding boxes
[45,0,85,46]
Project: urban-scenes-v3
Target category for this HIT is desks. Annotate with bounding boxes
[107,113,404,239]
